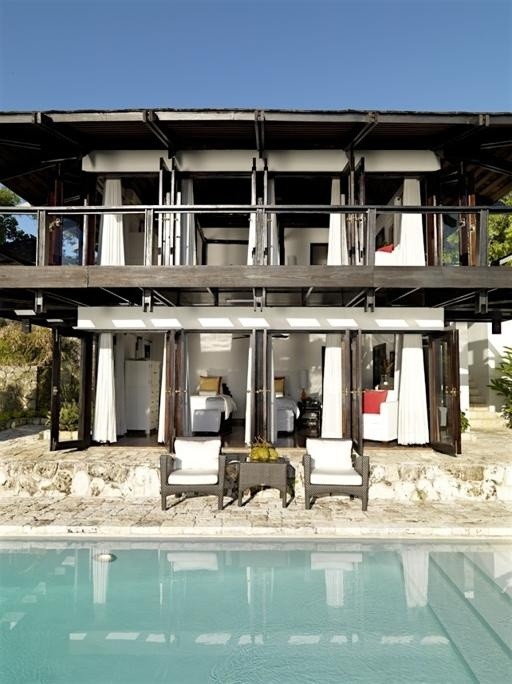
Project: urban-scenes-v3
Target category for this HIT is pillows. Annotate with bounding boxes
[197,376,225,396]
[273,376,287,397]
[363,386,388,415]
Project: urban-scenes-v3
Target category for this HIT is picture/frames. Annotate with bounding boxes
[375,226,385,251]
[390,350,395,377]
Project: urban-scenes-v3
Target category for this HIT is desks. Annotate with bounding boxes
[236,457,288,508]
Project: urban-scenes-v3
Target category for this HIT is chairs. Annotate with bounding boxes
[305,439,371,512]
[303,549,369,580]
[361,386,401,447]
[161,549,227,580]
[157,436,227,512]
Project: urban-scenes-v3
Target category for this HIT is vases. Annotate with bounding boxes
[378,373,391,390]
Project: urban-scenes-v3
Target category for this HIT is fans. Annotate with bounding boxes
[230,332,289,340]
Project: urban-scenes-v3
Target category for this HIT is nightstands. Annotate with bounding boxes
[296,400,322,431]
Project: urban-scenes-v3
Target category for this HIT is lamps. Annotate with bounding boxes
[299,369,309,399]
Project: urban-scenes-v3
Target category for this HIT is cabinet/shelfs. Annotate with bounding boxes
[123,358,162,434]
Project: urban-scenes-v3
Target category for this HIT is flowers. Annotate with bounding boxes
[377,358,393,376]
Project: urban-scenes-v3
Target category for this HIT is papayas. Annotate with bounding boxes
[252,447,278,460]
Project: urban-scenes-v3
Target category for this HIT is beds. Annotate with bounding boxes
[274,397,301,434]
[187,382,238,433]
